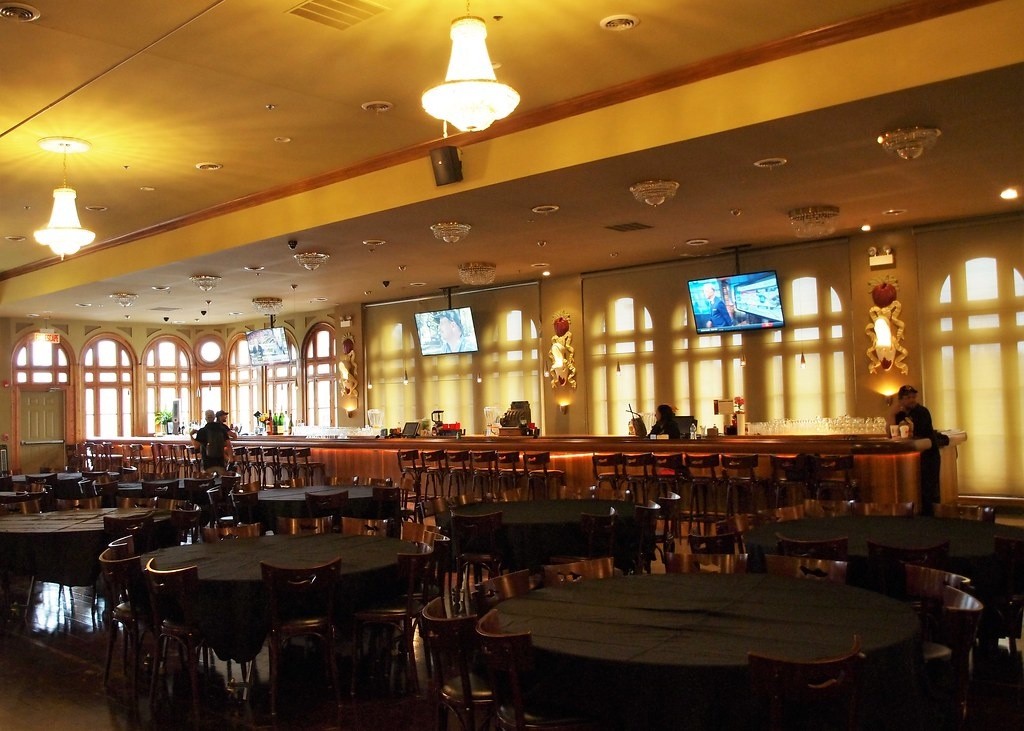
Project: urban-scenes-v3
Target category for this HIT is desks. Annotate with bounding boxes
[0,458,1024,731]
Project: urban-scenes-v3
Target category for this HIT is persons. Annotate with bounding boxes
[884,383,942,520]
[188,410,233,479]
[645,404,682,441]
[252,336,263,359]
[702,283,732,327]
[432,426,437,436]
[431,310,474,354]
[214,410,238,469]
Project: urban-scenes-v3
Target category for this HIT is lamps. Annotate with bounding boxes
[456,262,498,286]
[876,125,942,161]
[31,135,97,261]
[293,252,330,271]
[252,298,285,315]
[109,294,138,308]
[427,220,471,244]
[419,0,521,134]
[789,206,840,242]
[188,274,223,290]
[628,177,680,208]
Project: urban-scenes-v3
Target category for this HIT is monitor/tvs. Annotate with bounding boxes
[686,271,785,333]
[402,422,420,437]
[245,327,290,366]
[414,305,478,356]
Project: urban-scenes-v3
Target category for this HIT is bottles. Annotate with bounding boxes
[156,414,174,435]
[690,422,697,439]
[266,409,293,435]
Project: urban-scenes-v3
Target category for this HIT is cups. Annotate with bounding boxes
[700,425,706,439]
[900,425,909,438]
[299,421,374,440]
[891,424,899,436]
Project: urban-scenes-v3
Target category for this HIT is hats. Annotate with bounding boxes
[216,410,229,417]
[898,384,919,397]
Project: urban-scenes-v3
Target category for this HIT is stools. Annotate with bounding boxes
[396,446,862,556]
[70,438,327,500]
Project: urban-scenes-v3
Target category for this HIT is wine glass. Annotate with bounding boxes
[745,416,886,435]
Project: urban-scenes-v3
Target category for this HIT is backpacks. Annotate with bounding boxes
[202,426,225,459]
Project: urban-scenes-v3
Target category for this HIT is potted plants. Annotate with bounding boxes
[154,411,173,435]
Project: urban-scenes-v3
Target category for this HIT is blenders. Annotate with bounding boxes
[432,410,444,436]
